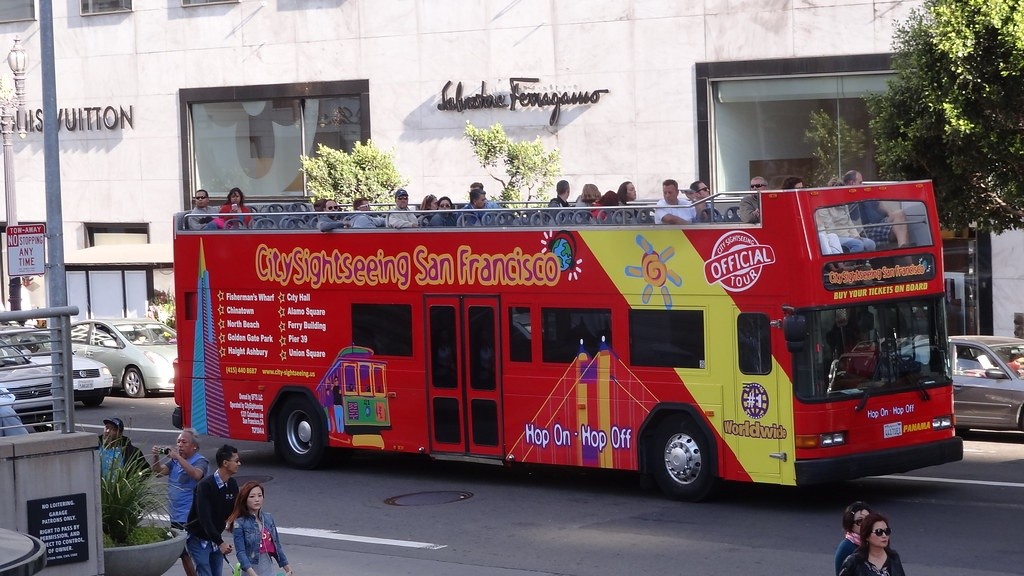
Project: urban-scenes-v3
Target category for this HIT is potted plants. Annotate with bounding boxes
[90,420,187,576]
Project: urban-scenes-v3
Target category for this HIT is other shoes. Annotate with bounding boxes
[900,242,917,248]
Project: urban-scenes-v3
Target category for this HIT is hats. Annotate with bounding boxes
[103,418,123,433]
[396,189,408,197]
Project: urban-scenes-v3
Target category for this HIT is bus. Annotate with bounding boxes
[173,178,963,501]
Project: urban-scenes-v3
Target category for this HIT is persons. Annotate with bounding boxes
[185,444,241,576]
[225,481,292,576]
[385,188,419,228]
[188,187,252,231]
[0,406,29,437]
[655,179,714,225]
[319,199,350,232]
[834,501,906,576]
[457,182,501,227]
[349,198,385,228]
[545,180,573,226]
[575,181,639,225]
[815,170,917,252]
[417,194,458,227]
[782,176,803,189]
[98,417,151,515]
[740,176,769,223]
[152,427,210,576]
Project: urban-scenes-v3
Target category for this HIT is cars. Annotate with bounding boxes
[0,325,113,407]
[0,339,52,432]
[894,335,1024,431]
[45,318,177,398]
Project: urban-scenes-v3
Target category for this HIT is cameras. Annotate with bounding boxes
[232,204,237,210]
[159,448,167,454]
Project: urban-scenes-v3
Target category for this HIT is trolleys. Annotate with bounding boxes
[224,544,242,576]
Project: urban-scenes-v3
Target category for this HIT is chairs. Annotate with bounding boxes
[219,202,323,230]
[418,204,751,223]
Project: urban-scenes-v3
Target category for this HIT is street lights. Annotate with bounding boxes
[0,34,29,310]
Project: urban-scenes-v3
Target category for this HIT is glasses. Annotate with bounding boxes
[396,197,408,200]
[853,519,863,526]
[325,206,337,210]
[871,527,891,536]
[751,184,767,188]
[358,204,369,208]
[439,204,451,208]
[1016,361,1024,365]
[477,198,486,202]
[196,196,207,199]
[695,186,709,194]
